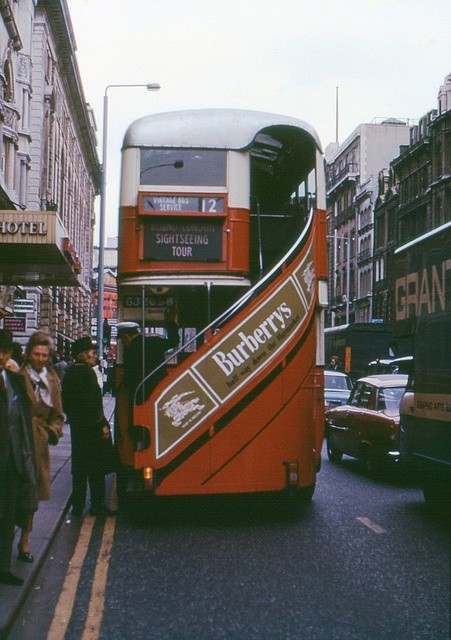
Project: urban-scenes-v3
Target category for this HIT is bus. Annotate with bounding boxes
[324,321,400,380]
[113,107,329,509]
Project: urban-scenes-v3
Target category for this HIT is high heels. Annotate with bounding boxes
[18,544,32,563]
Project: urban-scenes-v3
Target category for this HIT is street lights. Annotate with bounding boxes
[96,81,162,372]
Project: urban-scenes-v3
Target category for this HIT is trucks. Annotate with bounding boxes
[400,311,451,513]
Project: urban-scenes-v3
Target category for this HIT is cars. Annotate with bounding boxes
[325,373,355,411]
[324,373,409,480]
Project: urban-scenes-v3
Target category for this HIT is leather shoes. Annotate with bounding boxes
[72,508,81,516]
[90,504,117,516]
[0,572,23,584]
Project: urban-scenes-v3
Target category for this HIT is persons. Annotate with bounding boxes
[17,332,63,563]
[115,305,180,451]
[53,357,103,399]
[60,337,117,516]
[327,356,344,373]
[0,329,38,585]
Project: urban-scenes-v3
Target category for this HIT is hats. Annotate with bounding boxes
[115,322,139,337]
[0,329,17,346]
[71,337,99,358]
